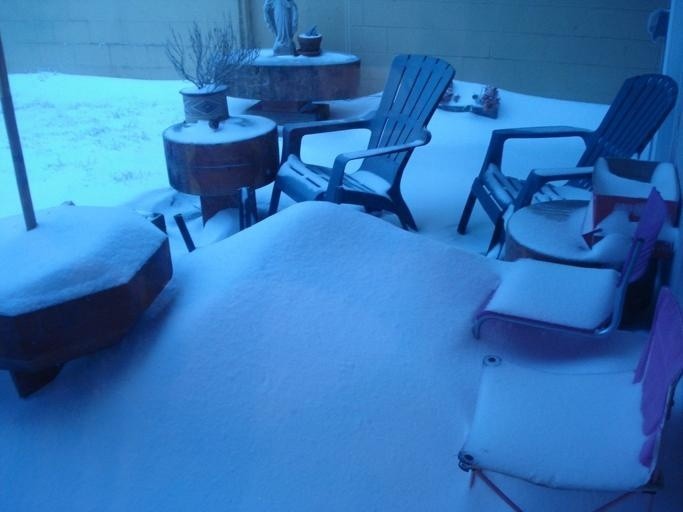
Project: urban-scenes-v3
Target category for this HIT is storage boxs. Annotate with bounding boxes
[591,159,678,233]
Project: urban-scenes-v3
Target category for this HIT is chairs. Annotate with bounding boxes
[458,285,683,512]
[269,53,456,234]
[472,186,668,339]
[457,74,679,260]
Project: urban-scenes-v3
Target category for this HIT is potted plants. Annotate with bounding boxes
[161,16,260,122]
